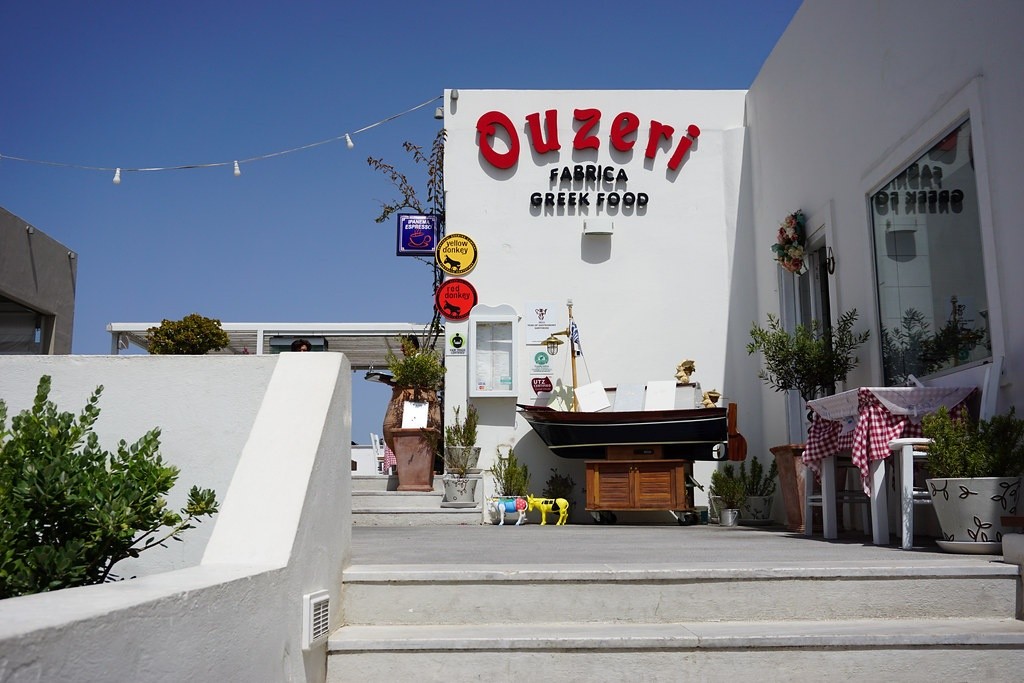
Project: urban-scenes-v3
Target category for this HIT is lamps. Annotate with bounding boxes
[541,328,570,355]
[451,89,459,100]
[584,219,614,234]
[25,226,33,234]
[887,218,917,233]
[434,107,444,119]
[68,251,74,259]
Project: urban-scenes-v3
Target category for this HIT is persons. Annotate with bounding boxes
[291,339,312,351]
[399,335,421,360]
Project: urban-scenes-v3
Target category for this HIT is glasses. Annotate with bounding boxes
[296,349,308,351]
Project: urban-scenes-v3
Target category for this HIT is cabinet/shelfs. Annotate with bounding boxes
[585,459,689,512]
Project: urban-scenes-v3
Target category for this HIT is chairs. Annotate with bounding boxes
[370,433,384,474]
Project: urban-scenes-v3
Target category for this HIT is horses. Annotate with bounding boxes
[485,494,569,526]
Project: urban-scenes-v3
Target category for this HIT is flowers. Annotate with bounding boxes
[771,214,809,277]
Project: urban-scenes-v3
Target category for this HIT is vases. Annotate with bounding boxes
[389,427,441,492]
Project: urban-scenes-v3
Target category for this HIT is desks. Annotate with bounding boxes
[802,386,981,545]
[384,444,397,475]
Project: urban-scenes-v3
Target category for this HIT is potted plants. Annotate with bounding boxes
[490,447,532,517]
[446,403,481,468]
[383,323,447,475]
[920,404,1024,542]
[724,456,778,520]
[718,477,746,527]
[709,471,738,519]
[542,468,576,524]
[746,309,871,533]
[418,426,478,503]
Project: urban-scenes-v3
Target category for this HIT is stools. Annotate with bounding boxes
[804,449,872,536]
[888,438,935,551]
